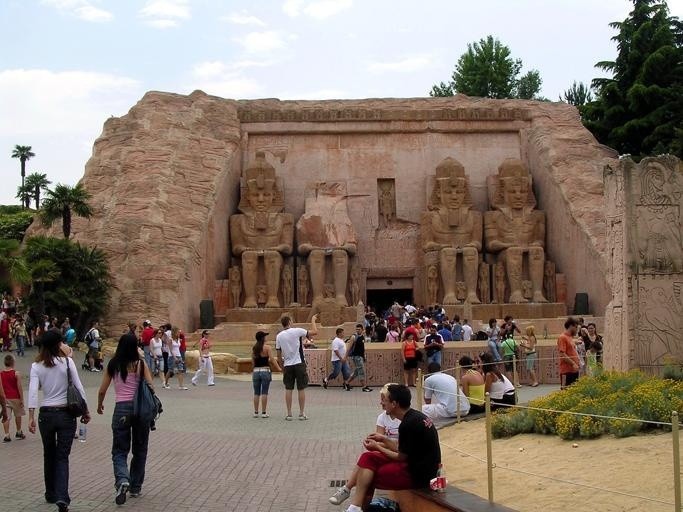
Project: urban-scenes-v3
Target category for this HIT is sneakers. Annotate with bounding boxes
[2,437,12,444]
[361,386,373,392]
[115,481,130,506]
[262,414,270,418]
[342,382,350,391]
[14,432,26,440]
[163,382,170,390]
[329,485,351,504]
[343,503,364,512]
[57,501,69,512]
[130,492,145,499]
[298,415,309,421]
[322,378,328,389]
[343,387,352,391]
[532,383,538,386]
[514,384,521,388]
[253,412,259,418]
[285,416,292,421]
[90,368,100,372]
[179,386,188,390]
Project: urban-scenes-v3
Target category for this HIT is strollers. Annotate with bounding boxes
[81,340,104,371]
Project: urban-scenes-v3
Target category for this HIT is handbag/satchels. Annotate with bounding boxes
[505,342,520,361]
[133,361,164,432]
[413,340,423,363]
[64,357,85,418]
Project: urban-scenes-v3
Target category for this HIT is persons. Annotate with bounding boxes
[422,363,471,417]
[61,317,71,338]
[451,316,462,341]
[484,158,545,303]
[501,373,515,408]
[478,352,504,412]
[361,301,451,329]
[486,318,502,361]
[0,291,15,353]
[462,319,473,341]
[438,324,451,341]
[586,342,603,377]
[0,375,8,423]
[424,325,445,373]
[583,323,603,362]
[500,316,520,340]
[363,384,441,512]
[95,349,104,370]
[15,319,28,356]
[140,319,155,374]
[276,314,318,421]
[520,326,538,387]
[401,333,419,387]
[323,329,353,390]
[343,325,373,392]
[48,316,59,333]
[420,156,483,304]
[26,340,28,341]
[557,318,580,389]
[329,382,402,512]
[0,355,26,441]
[384,325,399,342]
[85,322,100,373]
[28,330,91,512]
[15,291,28,319]
[400,319,421,342]
[578,318,588,328]
[39,315,48,338]
[497,332,522,388]
[459,356,485,413]
[150,329,166,388]
[164,328,188,390]
[126,321,137,334]
[374,321,388,342]
[97,334,154,505]
[230,167,294,308]
[576,326,588,350]
[191,330,215,386]
[252,331,282,418]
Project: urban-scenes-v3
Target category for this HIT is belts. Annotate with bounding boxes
[252,368,271,373]
[38,406,68,414]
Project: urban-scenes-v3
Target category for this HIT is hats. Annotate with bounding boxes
[255,331,269,339]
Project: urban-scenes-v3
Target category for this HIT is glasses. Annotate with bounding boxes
[204,332,208,335]
[157,332,162,334]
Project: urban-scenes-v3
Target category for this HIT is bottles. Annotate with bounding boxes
[78,420,86,442]
[436,464,446,493]
